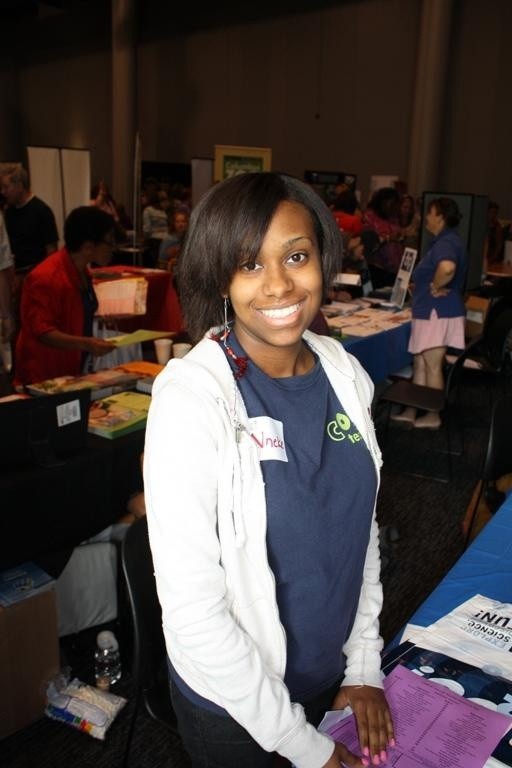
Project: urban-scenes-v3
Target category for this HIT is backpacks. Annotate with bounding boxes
[54,523,132,698]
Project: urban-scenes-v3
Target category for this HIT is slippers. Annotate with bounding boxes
[390,418,443,431]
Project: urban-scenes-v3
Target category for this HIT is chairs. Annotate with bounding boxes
[464,393,512,548]
[374,335,484,479]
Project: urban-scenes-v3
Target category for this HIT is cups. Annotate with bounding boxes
[153,338,193,366]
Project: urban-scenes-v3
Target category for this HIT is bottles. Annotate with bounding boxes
[93,629,124,692]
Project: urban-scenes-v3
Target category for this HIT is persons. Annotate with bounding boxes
[0,151,512,438]
[139,167,401,767]
